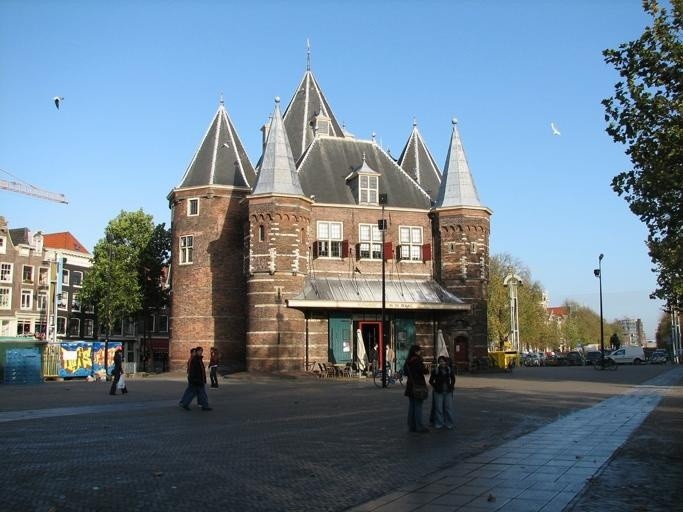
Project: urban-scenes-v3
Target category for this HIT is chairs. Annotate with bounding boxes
[422,356,464,376]
[318,362,352,380]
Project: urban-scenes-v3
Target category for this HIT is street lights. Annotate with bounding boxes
[594,254,603,367]
[428,207,437,365]
[104,233,117,371]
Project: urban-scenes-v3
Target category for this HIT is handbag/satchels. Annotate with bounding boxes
[412,386,429,400]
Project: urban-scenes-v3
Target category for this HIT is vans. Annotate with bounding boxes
[604,348,645,366]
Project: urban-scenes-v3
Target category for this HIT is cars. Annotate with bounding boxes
[519,352,582,366]
[652,348,667,364]
[585,352,601,365]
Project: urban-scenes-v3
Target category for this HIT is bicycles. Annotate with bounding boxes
[595,355,617,370]
[374,363,404,387]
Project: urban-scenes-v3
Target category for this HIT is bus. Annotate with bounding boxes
[488,351,517,368]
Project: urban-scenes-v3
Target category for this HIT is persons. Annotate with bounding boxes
[179,347,219,411]
[386,344,396,377]
[369,343,379,378]
[109,350,128,395]
[403,346,430,432]
[429,356,455,429]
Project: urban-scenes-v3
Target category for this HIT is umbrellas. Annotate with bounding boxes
[436,330,450,368]
[356,328,368,378]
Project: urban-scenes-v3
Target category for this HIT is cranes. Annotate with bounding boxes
[0,169,68,204]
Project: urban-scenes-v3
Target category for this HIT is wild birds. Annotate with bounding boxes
[550,122,562,137]
[50,95,64,110]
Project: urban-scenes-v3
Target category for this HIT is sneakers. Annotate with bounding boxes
[208,384,219,390]
[408,421,456,434]
[178,401,214,411]
